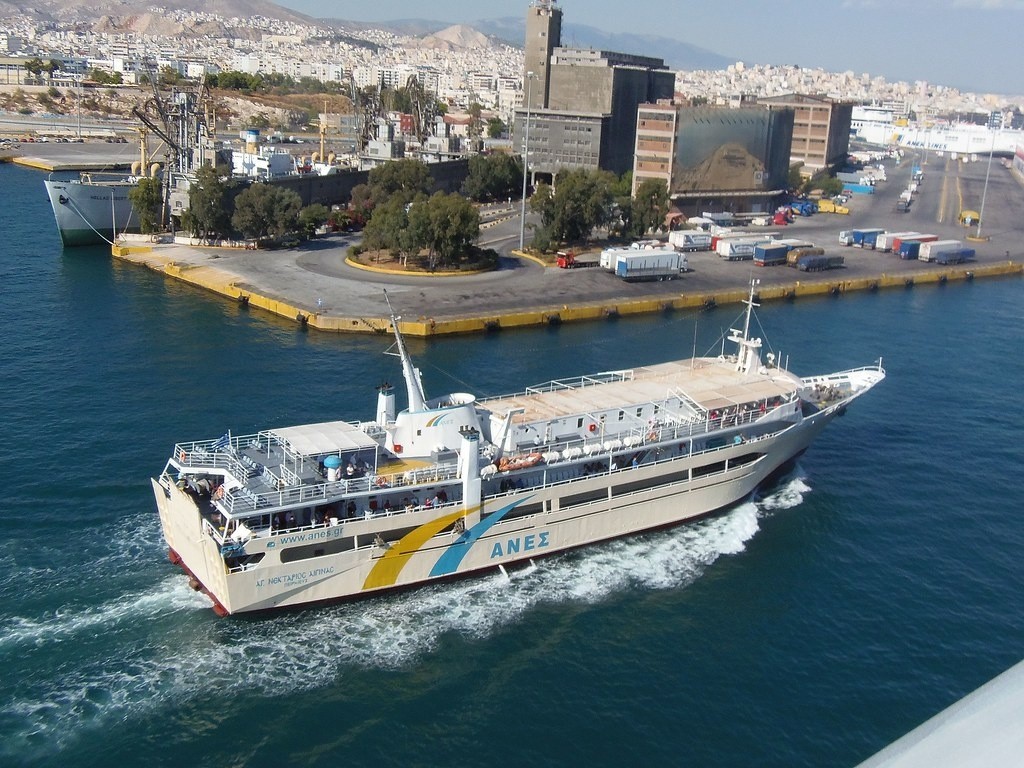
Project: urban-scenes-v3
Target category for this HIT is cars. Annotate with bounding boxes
[1,136,327,144]
[896,169,923,215]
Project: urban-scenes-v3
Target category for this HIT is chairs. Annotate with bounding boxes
[189,439,287,507]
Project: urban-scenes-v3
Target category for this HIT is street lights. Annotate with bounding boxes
[965,126,1002,242]
[518,70,542,252]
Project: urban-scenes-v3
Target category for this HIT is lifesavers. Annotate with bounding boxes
[831,288,841,296]
[608,310,619,322]
[485,322,500,332]
[377,476,386,485]
[967,273,974,281]
[649,431,656,440]
[905,279,913,289]
[180,452,186,462]
[663,303,674,312]
[869,282,878,290]
[787,292,795,300]
[549,315,561,327]
[217,486,223,498]
[753,294,760,303]
[705,300,716,308]
[940,275,947,283]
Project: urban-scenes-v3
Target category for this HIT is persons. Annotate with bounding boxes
[733,432,745,444]
[533,434,540,452]
[500,478,525,492]
[633,457,638,468]
[347,453,370,479]
[274,514,280,529]
[315,507,332,523]
[583,462,604,476]
[384,499,391,512]
[336,465,342,481]
[348,501,356,518]
[425,495,438,509]
[286,510,296,528]
[680,444,687,455]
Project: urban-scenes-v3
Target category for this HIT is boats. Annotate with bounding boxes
[493,452,542,472]
[42,72,523,248]
[149,279,887,617]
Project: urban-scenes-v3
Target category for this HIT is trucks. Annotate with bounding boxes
[838,228,976,266]
[599,163,887,283]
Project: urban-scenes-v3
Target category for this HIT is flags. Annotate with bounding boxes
[211,431,230,450]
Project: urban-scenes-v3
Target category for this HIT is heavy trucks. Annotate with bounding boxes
[556,248,598,269]
